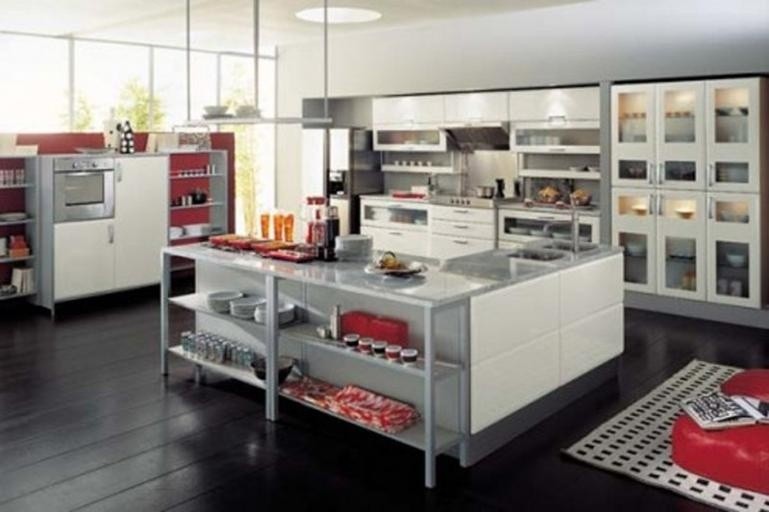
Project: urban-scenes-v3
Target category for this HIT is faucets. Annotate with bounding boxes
[568,191,582,253]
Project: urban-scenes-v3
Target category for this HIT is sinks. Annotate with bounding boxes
[550,240,597,254]
[513,244,565,263]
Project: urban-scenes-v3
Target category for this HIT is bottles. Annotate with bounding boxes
[179,328,253,367]
[330,304,342,340]
[103,105,135,154]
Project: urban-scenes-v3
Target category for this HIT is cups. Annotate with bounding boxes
[259,211,294,241]
[0,168,25,186]
[393,159,433,166]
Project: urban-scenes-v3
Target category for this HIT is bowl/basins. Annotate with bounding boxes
[188,191,208,203]
[250,355,297,384]
[632,203,648,215]
[170,222,213,240]
[674,206,696,220]
[570,165,600,172]
[626,241,645,258]
[203,104,229,115]
[724,249,746,268]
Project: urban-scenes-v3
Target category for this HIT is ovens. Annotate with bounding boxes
[52,154,115,223]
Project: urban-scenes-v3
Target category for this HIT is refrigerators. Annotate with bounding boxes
[299,128,385,238]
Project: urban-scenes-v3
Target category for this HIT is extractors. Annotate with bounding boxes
[436,123,510,153]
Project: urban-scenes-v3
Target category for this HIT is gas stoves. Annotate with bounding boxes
[436,189,514,201]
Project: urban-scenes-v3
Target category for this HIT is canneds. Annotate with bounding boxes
[180,330,254,368]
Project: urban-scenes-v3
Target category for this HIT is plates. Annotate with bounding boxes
[505,223,592,243]
[363,258,429,279]
[203,288,297,327]
[0,210,28,222]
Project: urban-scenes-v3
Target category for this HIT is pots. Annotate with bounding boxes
[475,184,494,197]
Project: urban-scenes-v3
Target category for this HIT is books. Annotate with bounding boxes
[680,388,769,431]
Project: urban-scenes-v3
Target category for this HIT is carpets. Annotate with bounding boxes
[558,356,769,512]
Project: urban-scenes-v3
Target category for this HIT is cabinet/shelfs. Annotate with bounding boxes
[159,230,625,489]
[0,128,234,325]
[304,71,769,328]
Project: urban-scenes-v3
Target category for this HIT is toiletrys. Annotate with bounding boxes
[259,213,271,238]
[274,215,284,241]
[284,215,294,241]
